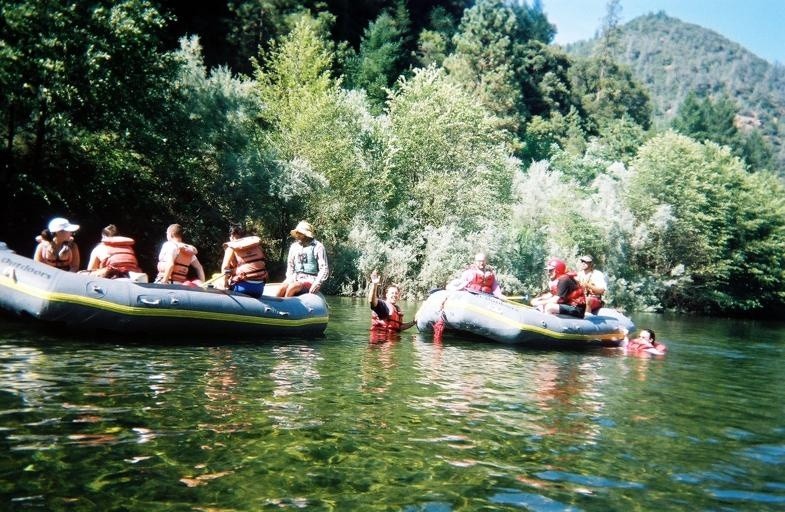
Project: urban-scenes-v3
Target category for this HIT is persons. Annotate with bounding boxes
[34,218,80,272]
[222,225,269,299]
[87,224,141,279]
[629,329,666,355]
[574,254,607,315]
[277,220,330,300]
[532,259,585,317]
[154,223,206,287]
[445,252,508,301]
[368,269,417,343]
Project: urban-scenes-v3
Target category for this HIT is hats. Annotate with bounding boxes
[579,255,593,262]
[290,221,314,239]
[129,271,148,283]
[48,217,81,232]
[475,254,485,261]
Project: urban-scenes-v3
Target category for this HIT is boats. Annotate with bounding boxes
[0,242,330,342]
[415,287,636,347]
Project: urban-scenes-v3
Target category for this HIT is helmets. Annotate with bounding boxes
[544,260,566,274]
